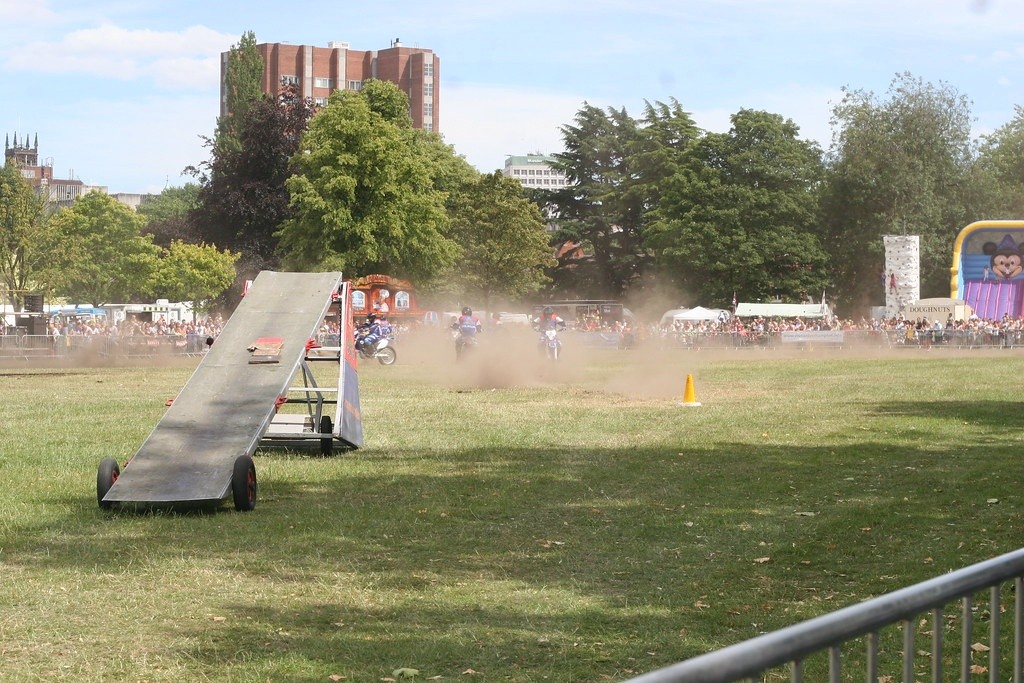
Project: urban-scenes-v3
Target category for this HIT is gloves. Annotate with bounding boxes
[476,326,482,333]
[535,328,541,333]
[561,327,567,332]
[453,322,460,329]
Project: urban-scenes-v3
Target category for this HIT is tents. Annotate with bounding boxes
[674,306,730,329]
[734,302,830,323]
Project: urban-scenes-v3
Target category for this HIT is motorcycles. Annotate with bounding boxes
[451,323,483,372]
[340,328,397,366]
[538,327,564,364]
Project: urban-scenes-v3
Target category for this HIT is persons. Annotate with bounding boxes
[378,315,392,340]
[451,306,485,366]
[47,312,222,354]
[314,318,425,348]
[356,312,383,357]
[531,305,567,360]
[572,313,1024,348]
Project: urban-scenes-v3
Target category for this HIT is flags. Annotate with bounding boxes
[732,292,737,306]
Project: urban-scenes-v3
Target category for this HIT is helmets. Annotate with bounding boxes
[368,313,376,319]
[380,316,386,320]
[462,306,472,315]
[544,307,554,314]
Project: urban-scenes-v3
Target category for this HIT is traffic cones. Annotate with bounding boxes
[680,372,702,407]
